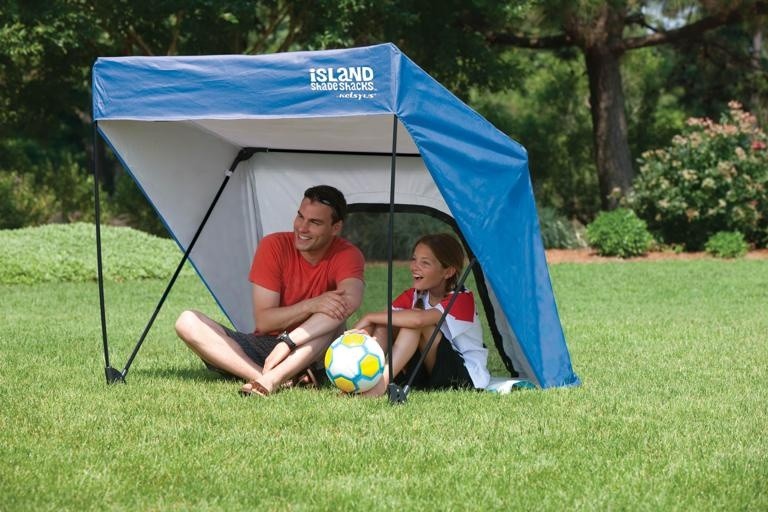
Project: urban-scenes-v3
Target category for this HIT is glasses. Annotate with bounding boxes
[303,187,348,222]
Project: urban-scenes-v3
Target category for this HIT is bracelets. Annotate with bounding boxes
[276,331,296,351]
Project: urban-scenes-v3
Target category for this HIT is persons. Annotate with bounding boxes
[351,232,491,398]
[175,184,366,398]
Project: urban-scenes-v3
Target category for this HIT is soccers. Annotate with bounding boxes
[325,332,385,394]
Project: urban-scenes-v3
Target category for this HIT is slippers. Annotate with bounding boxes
[237,378,271,401]
[285,367,319,389]
